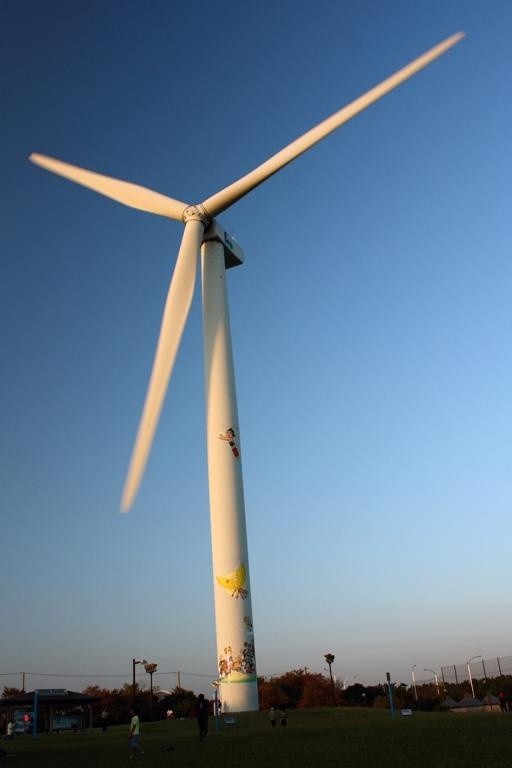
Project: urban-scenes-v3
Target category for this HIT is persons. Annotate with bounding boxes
[191,694,209,741]
[3,718,17,741]
[279,708,288,726]
[268,705,277,727]
[506,688,512,712]
[498,688,509,713]
[100,706,110,732]
[215,702,222,732]
[158,706,181,721]
[127,704,145,760]
[21,712,31,721]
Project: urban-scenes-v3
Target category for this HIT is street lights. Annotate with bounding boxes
[144,662,157,704]
[423,666,442,694]
[324,653,337,707]
[467,654,484,699]
[411,663,419,700]
[132,659,147,702]
[386,672,395,720]
